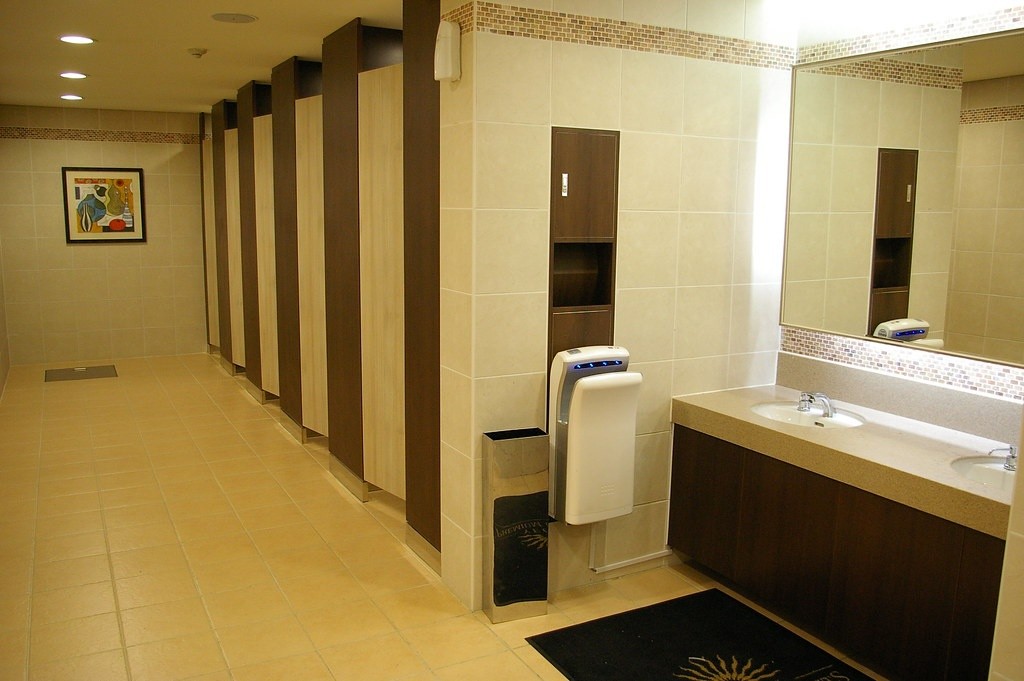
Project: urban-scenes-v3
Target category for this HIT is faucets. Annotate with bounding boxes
[808,393,832,418]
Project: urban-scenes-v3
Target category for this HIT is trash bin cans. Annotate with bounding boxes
[482,428,551,624]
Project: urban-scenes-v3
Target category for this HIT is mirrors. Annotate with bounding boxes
[777,27,1024,368]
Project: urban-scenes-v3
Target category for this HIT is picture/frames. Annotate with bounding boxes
[61,169,147,243]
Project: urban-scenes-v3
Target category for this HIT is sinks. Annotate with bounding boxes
[750,400,867,429]
[950,456,1018,490]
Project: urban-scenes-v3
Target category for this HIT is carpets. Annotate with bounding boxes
[524,589,880,681]
